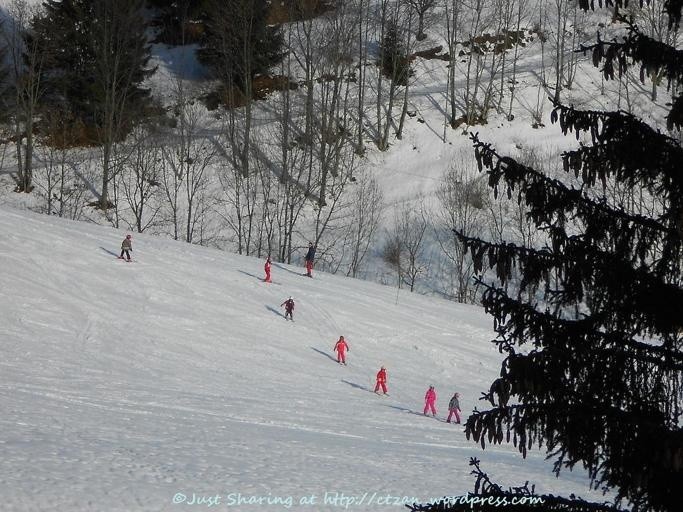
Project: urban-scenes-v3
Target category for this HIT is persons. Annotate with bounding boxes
[334,335,350,364]
[303,243,315,278]
[423,385,437,417]
[264,257,272,284]
[447,392,462,424]
[281,297,295,321]
[374,366,389,395]
[118,234,133,262]
